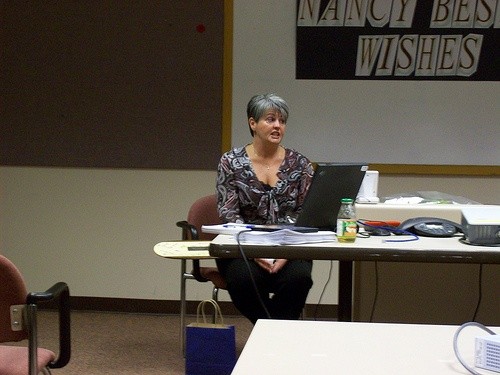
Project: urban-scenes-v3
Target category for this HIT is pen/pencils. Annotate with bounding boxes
[187,245,208,251]
[223,225,255,228]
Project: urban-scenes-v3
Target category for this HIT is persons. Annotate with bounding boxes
[215,93,314,325]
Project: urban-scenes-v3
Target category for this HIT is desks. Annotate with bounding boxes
[232,319,500,375]
[209,232,500,322]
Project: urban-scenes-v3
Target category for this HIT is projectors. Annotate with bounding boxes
[460,205,500,247]
[474,334,500,373]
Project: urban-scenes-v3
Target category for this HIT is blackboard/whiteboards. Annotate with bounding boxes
[0,0,223,171]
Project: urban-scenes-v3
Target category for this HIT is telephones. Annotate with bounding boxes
[395,215,463,238]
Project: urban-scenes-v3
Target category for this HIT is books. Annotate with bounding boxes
[202,222,335,245]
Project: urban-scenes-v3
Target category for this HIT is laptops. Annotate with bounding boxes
[295,162,369,230]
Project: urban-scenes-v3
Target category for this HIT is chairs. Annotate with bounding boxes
[0,255,71,375]
[176,194,306,358]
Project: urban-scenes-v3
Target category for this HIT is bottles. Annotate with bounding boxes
[336,199,357,244]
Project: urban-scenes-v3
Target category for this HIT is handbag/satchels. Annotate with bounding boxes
[185,299,237,375]
